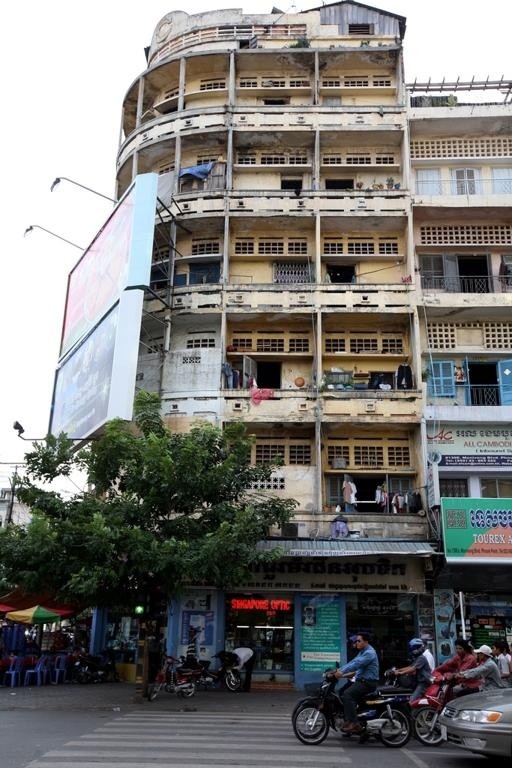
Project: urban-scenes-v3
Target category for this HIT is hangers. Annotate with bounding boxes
[400,358,408,366]
[343,477,355,484]
[392,487,420,496]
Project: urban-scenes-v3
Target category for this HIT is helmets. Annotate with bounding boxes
[408,638,425,656]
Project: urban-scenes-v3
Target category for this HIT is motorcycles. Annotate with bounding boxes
[292,661,460,746]
[180,649,241,691]
[148,652,205,701]
[72,644,120,684]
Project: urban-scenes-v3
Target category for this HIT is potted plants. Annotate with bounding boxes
[386,176,393,190]
[345,376,353,390]
[356,176,363,190]
[322,375,336,390]
[422,368,430,382]
[336,380,345,390]
[372,178,378,190]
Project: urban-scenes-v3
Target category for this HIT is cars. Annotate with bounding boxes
[432,686,512,763]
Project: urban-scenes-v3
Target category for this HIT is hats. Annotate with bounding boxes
[474,644,493,657]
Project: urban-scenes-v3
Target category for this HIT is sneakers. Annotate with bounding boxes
[341,722,362,733]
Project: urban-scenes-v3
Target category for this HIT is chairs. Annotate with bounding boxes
[0,650,82,687]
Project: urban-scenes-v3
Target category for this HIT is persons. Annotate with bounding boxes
[326,632,512,733]
[232,646,257,691]
[1,620,162,682]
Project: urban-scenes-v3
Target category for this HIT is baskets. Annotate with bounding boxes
[305,682,321,697]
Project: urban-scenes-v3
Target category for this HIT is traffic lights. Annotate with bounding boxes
[135,605,144,614]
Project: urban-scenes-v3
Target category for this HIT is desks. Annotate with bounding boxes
[352,376,371,389]
